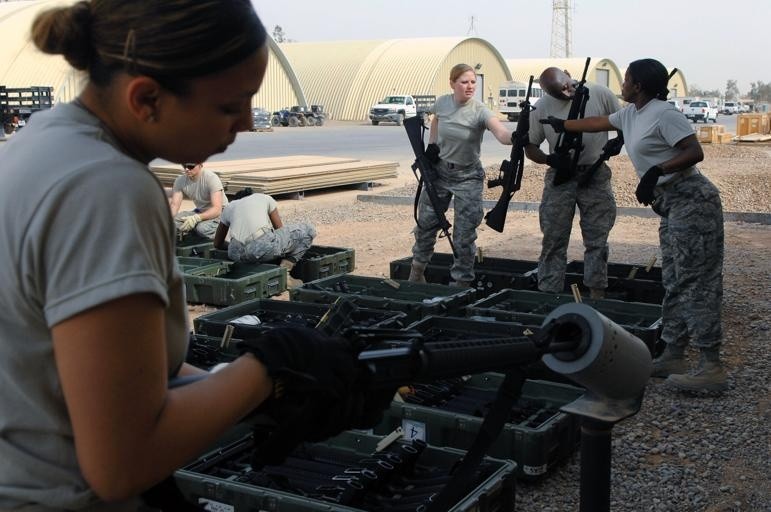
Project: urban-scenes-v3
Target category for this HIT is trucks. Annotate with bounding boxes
[0,85,55,142]
[368,95,436,126]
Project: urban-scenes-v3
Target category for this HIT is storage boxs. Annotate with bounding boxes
[697,110,771,145]
[174,234,665,511]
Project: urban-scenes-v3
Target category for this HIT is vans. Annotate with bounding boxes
[665,97,754,124]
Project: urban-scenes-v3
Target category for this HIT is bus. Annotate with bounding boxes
[497,80,548,121]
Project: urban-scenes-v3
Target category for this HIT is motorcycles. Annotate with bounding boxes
[247,104,330,129]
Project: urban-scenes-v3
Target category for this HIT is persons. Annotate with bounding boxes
[409,63,515,289]
[214,188,316,288]
[1,0,361,512]
[170,160,229,240]
[523,67,624,298]
[540,59,729,390]
[10,116,24,133]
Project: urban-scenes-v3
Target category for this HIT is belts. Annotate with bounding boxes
[439,159,467,170]
[232,228,268,248]
[653,166,698,198]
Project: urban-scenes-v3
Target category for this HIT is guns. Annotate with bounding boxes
[552,58,590,186]
[169,318,573,390]
[408,381,553,428]
[485,76,534,233]
[403,113,459,259]
[220,439,456,512]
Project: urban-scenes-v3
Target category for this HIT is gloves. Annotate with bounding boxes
[606,137,622,156]
[238,325,396,435]
[425,143,440,162]
[179,214,201,232]
[546,154,569,169]
[512,131,529,146]
[539,115,567,134]
[635,166,662,205]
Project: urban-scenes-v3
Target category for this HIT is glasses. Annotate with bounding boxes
[182,164,195,170]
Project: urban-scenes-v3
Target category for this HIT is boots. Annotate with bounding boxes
[668,349,729,393]
[407,262,426,283]
[279,259,303,286]
[651,344,688,377]
